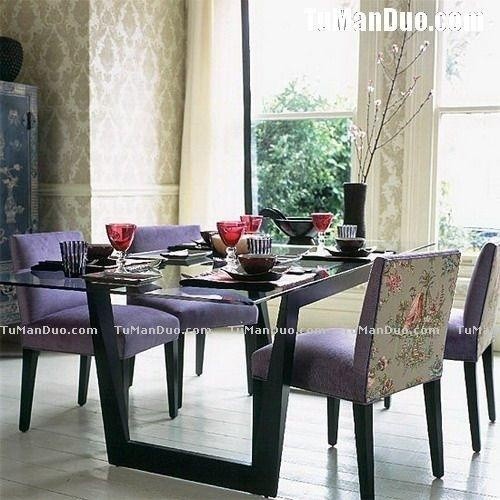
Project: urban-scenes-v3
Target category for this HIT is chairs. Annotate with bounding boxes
[127,224,257,406]
[9,230,180,431]
[444,241,500,453]
[251,249,461,500]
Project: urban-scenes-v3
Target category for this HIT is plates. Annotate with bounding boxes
[86,248,369,281]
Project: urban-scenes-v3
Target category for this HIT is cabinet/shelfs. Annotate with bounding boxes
[1,82,40,333]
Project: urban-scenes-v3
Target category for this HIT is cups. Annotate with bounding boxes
[59,225,358,278]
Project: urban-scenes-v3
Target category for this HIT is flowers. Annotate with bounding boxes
[348,1,441,183]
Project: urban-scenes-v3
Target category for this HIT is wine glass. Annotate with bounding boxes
[104,212,334,275]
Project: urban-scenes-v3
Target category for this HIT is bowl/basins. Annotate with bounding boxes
[87,229,364,274]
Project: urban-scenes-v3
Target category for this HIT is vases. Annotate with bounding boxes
[341,184,367,237]
